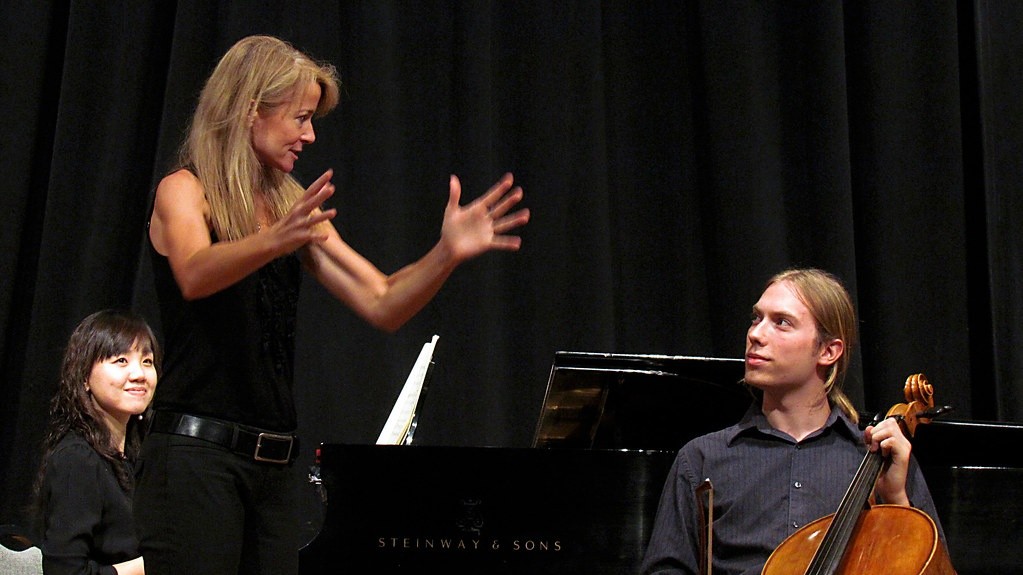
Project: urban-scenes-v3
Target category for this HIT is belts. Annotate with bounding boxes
[148,409,300,466]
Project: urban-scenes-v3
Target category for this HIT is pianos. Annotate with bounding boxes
[293,347,753,575]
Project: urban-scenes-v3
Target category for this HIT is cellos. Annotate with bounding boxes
[759,371,958,575]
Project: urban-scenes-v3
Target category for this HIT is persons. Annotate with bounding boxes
[29,308,163,575]
[638,268,951,575]
[130,36,528,575]
